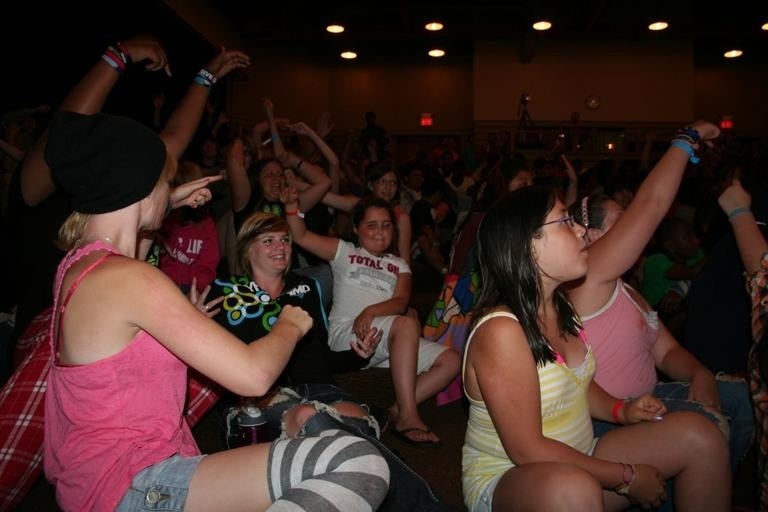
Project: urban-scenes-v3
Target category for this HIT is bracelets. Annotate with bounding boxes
[725,206,750,220]
[100,42,132,74]
[612,459,635,497]
[611,395,623,425]
[193,67,216,91]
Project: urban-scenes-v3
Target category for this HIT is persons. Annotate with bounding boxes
[216,211,446,512]
[715,166,767,511]
[40,107,390,512]
[0,96,51,209]
[142,83,766,447]
[458,180,732,512]
[0,34,250,512]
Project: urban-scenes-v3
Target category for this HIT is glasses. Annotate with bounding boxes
[532,212,575,234]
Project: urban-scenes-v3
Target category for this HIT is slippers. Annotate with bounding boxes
[388,419,442,448]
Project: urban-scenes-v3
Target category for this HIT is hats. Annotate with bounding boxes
[43,109,167,214]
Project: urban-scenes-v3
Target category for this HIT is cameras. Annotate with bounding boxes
[519,91,530,105]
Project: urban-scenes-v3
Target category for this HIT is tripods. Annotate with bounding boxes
[517,105,536,130]
